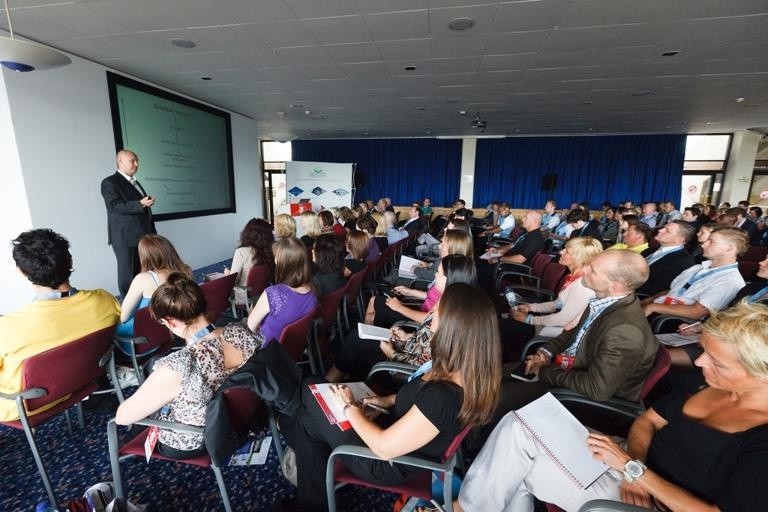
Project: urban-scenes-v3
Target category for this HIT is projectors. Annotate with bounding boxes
[471,121,487,128]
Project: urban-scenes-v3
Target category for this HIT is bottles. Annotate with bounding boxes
[503,285,520,314]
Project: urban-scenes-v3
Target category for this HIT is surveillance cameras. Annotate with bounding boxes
[460,110,467,116]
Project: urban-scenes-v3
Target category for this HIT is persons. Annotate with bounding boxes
[114,272,266,459]
[115,233,194,388]
[325,200,768,458]
[414,294,767,512]
[101,151,157,306]
[1,228,121,421]
[282,282,502,512]
[199,196,433,367]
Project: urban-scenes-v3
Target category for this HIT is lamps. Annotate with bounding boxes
[0,1,73,74]
[267,111,299,145]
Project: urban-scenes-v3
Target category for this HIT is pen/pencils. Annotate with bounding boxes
[677,320,702,332]
[391,287,400,294]
[358,399,390,414]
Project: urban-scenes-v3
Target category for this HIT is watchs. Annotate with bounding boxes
[624,459,647,483]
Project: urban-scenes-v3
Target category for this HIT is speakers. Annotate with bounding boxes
[542,175,558,191]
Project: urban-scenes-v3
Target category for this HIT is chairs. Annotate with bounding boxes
[1,212,768,511]
